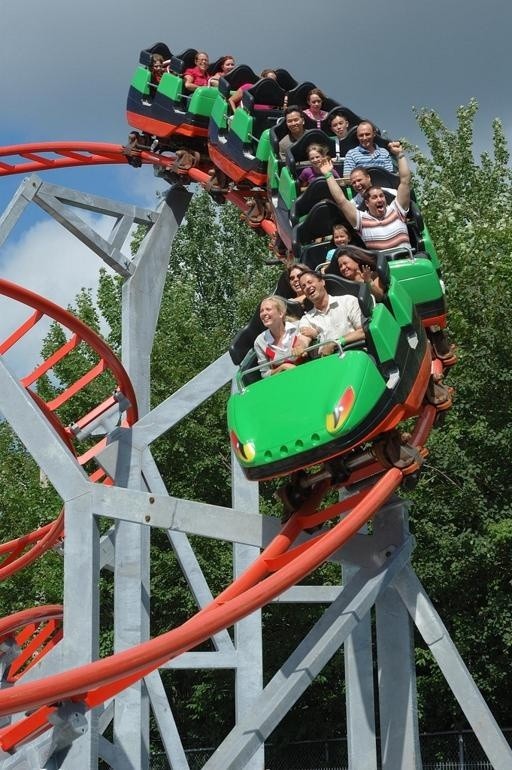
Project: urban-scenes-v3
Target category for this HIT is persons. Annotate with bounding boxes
[252,294,307,380]
[341,120,395,184]
[323,223,352,263]
[326,246,395,317]
[145,39,353,161]
[344,168,386,207]
[291,269,368,359]
[316,139,413,261]
[282,263,313,310]
[295,144,342,193]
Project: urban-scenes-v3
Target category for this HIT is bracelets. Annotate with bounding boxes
[396,153,406,160]
[324,172,333,182]
[336,336,347,349]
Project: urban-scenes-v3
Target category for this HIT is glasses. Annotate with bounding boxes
[288,273,302,282]
